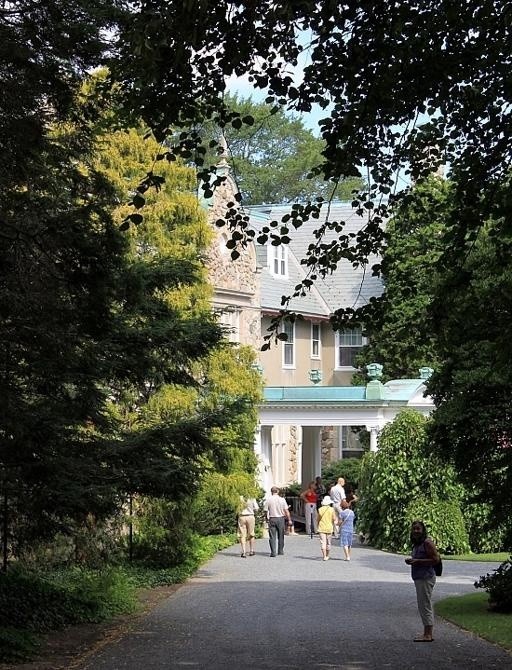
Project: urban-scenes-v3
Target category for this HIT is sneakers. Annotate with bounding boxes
[323,556,329,561]
[413,635,435,642]
[240,552,256,558]
[344,555,352,561]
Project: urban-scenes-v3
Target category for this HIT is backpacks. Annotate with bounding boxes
[423,539,443,576]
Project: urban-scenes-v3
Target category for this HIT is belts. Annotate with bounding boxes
[240,514,254,517]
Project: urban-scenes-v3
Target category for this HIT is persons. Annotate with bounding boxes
[299,476,355,561]
[264,486,292,558]
[405,521,437,641]
[236,493,260,558]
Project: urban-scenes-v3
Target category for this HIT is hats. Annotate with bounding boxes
[320,495,334,505]
[339,498,351,507]
